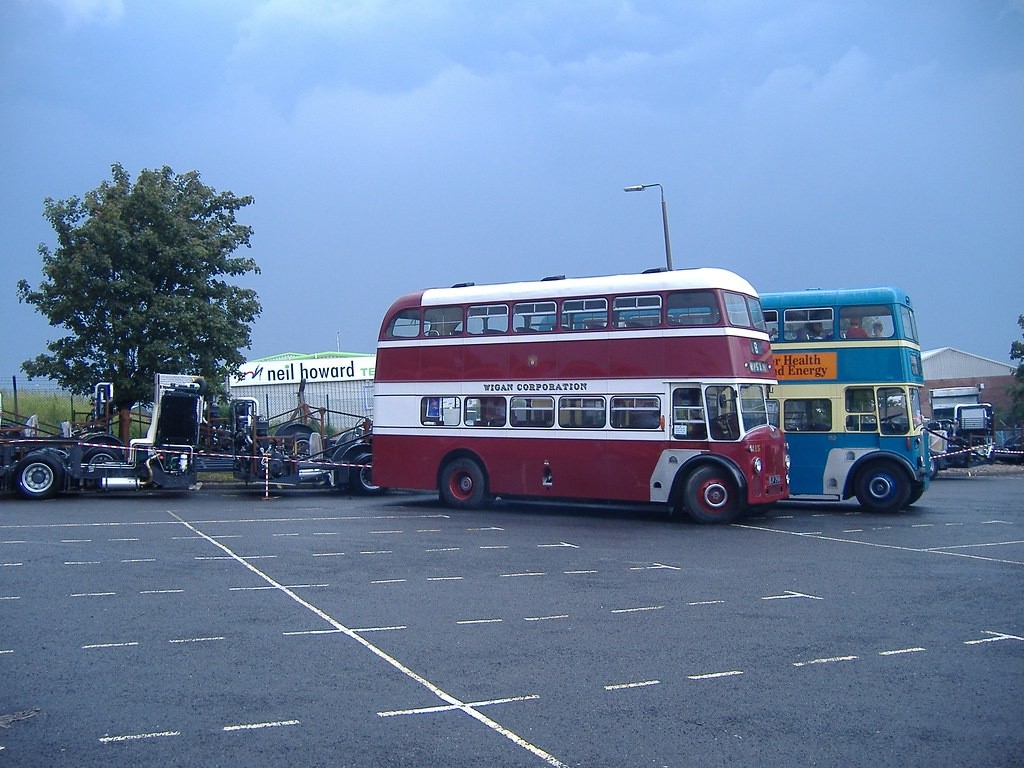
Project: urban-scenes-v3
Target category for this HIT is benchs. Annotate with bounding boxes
[424,320,681,337]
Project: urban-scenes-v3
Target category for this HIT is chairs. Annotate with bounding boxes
[771,332,846,343]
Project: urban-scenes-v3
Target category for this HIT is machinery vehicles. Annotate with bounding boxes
[925,402,996,476]
[0,374,390,500]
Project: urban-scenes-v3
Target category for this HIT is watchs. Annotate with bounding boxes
[492,416,493,419]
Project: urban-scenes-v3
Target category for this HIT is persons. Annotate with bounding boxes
[872,318,883,335]
[486,398,505,426]
[847,317,868,338]
[805,322,823,340]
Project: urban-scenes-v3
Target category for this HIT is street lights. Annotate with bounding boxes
[622,183,672,271]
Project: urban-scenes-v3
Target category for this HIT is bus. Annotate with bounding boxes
[371,266,790,524]
[538,286,932,516]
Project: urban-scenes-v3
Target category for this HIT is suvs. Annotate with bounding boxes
[1004,436,1024,452]
